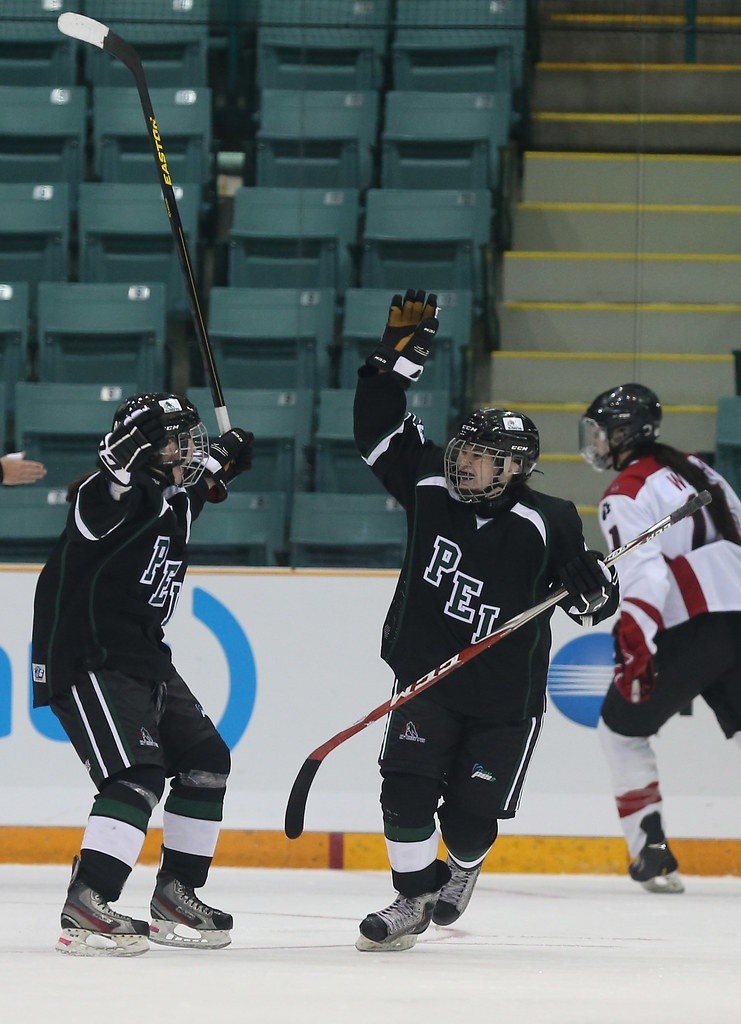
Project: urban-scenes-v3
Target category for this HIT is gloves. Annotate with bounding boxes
[546,550,613,615]
[98,396,170,488]
[610,620,657,705]
[364,287,439,388]
[189,427,255,503]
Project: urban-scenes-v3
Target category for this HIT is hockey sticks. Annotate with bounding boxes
[55,8,236,438]
[283,488,715,842]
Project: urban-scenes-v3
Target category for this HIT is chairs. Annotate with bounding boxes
[0,0,533,565]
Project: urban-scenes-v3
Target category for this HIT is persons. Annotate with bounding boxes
[30,391,253,959]
[576,382,741,894]
[0,451,47,488]
[353,288,619,951]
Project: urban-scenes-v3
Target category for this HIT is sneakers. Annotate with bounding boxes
[149,869,233,950]
[55,855,149,957]
[356,890,440,951]
[431,853,486,926]
[629,839,684,894]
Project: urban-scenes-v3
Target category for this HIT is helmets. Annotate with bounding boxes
[444,406,540,503]
[108,392,210,488]
[578,382,663,472]
